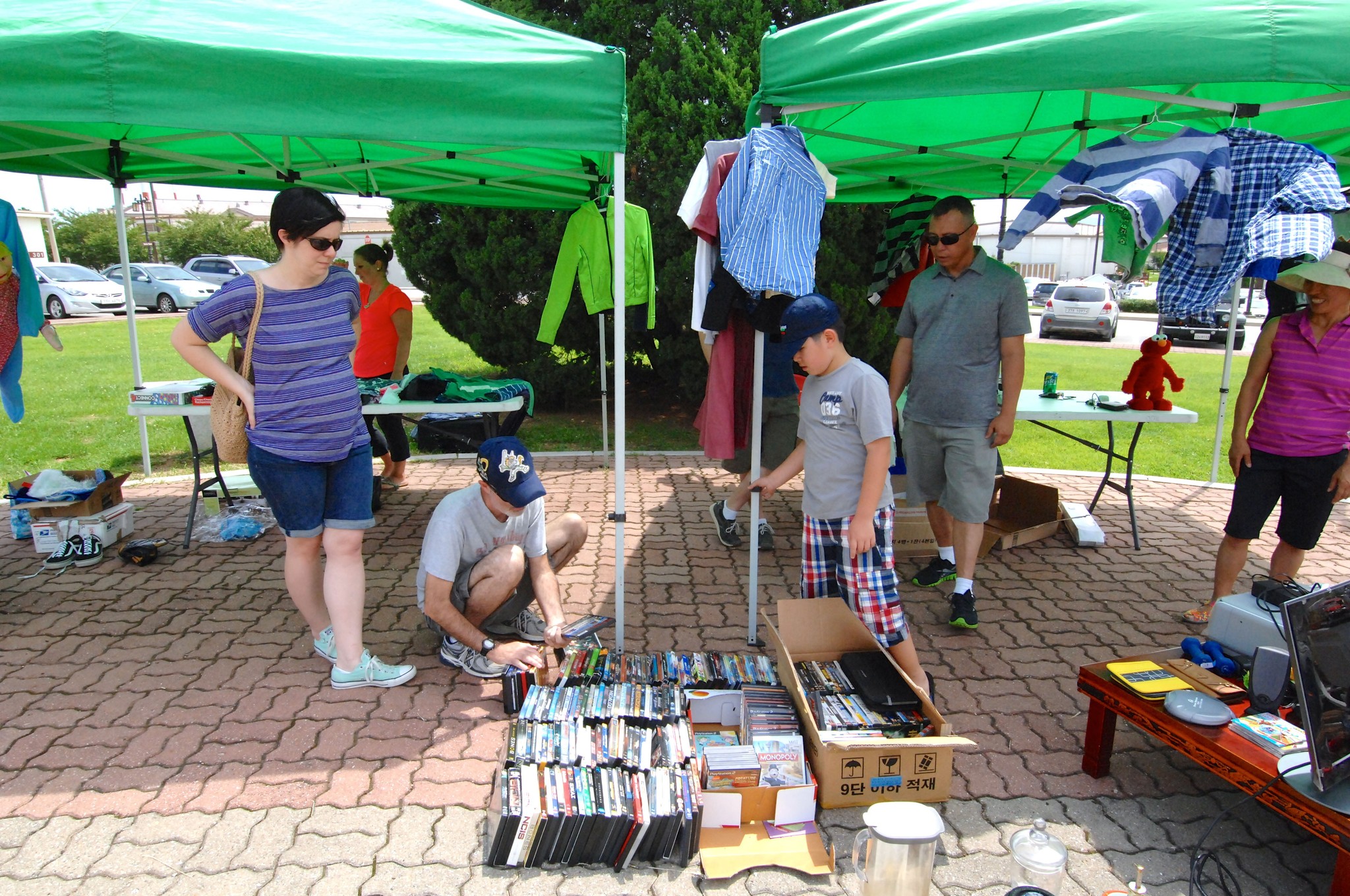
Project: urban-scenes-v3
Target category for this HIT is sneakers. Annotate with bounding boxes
[440,634,506,677]
[75,534,104,567]
[947,588,978,629]
[314,625,338,664]
[758,523,776,550]
[709,499,742,547]
[17,534,80,579]
[912,552,957,588]
[485,604,547,641]
[331,649,417,689]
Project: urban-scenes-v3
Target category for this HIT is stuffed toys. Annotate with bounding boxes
[1121,335,1185,411]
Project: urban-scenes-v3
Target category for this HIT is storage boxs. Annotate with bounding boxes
[31,503,134,553]
[891,475,938,550]
[760,596,977,809]
[129,381,212,405]
[694,722,835,880]
[977,475,1106,558]
[202,474,270,516]
[8,470,132,518]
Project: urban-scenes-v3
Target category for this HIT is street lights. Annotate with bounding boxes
[132,194,155,263]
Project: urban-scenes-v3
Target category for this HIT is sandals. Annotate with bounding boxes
[1184,600,1217,623]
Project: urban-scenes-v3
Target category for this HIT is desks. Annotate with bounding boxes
[895,387,1198,550]
[1077,642,1350,896]
[127,395,523,548]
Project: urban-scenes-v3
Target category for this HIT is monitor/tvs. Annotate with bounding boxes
[1279,580,1350,816]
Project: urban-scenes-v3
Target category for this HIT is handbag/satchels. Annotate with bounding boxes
[209,270,264,464]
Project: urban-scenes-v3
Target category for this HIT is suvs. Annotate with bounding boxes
[184,255,272,288]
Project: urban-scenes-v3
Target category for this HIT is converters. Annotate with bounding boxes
[1251,580,1304,605]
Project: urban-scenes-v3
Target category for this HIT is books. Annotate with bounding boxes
[1228,712,1308,759]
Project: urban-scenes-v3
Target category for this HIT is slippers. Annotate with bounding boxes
[379,474,386,479]
[381,480,408,490]
[924,671,935,704]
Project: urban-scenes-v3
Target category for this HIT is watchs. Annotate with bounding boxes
[480,637,495,656]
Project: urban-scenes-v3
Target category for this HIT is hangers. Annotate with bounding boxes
[1124,93,1184,135]
[593,174,612,212]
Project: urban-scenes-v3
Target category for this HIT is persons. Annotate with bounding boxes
[1184,238,1350,623]
[747,297,935,706]
[889,195,1032,627]
[416,437,587,677]
[709,285,820,549]
[171,187,417,690]
[353,242,414,490]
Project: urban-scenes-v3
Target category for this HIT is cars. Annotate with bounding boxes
[1021,277,1120,342]
[1157,289,1246,351]
[9,262,136,319]
[1116,280,1158,300]
[98,262,221,313]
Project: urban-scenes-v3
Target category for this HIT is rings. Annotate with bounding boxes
[546,636,550,639]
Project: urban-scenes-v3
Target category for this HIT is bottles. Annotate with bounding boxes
[1009,818,1068,896]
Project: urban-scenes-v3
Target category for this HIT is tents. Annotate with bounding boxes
[0,0,627,651]
[747,0,1350,647]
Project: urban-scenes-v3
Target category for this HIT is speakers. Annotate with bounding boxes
[1245,645,1293,719]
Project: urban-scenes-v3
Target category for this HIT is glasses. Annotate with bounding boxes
[299,236,343,250]
[925,220,976,246]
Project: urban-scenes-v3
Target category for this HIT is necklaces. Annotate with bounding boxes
[364,280,387,308]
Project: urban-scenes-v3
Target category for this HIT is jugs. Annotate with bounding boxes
[852,801,945,896]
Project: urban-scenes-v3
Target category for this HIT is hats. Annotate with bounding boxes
[1273,250,1350,292]
[777,294,839,361]
[476,436,547,508]
